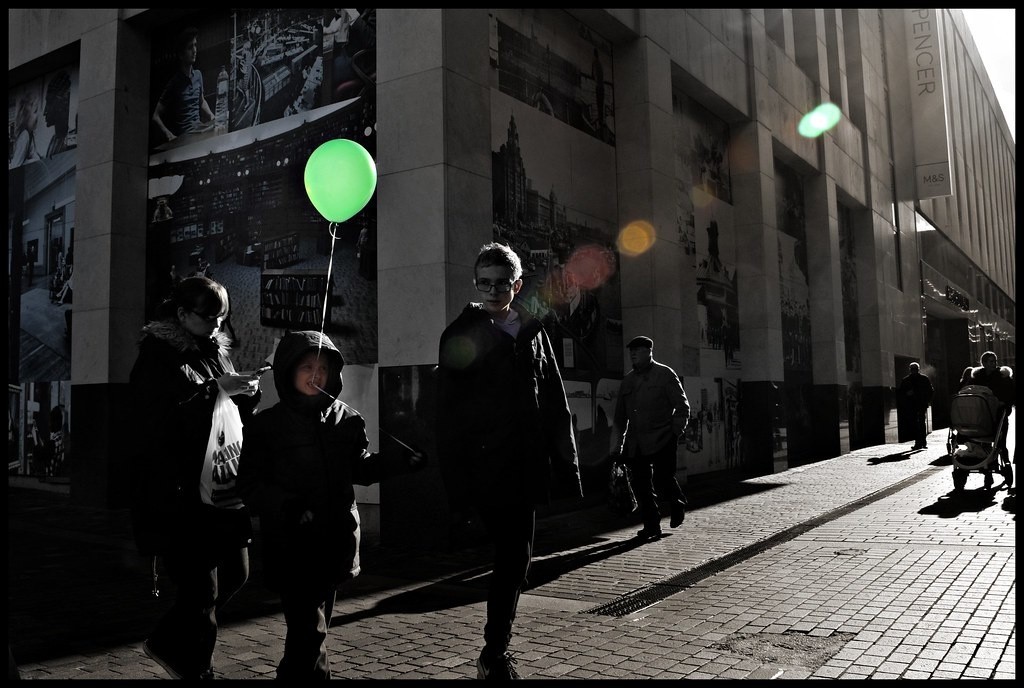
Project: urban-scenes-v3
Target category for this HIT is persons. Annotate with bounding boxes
[900,362,934,450]
[43,68,78,159]
[9,75,41,169]
[220,288,239,348]
[430,241,584,680]
[959,351,1017,464]
[127,276,260,678]
[152,31,229,140]
[357,223,369,247]
[27,246,73,304]
[608,336,691,536]
[237,8,377,117]
[238,330,421,679]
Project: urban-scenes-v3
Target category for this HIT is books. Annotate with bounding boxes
[264,235,300,264]
[262,276,326,324]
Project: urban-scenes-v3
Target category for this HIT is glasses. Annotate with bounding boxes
[476,281,511,293]
[176,304,225,323]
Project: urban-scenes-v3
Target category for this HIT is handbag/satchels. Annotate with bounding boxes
[199,382,246,509]
[612,460,638,512]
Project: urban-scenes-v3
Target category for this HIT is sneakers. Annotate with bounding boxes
[477,647,520,679]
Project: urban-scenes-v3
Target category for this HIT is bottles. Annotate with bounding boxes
[216,65,228,123]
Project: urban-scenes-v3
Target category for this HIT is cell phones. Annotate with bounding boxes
[250,365,271,379]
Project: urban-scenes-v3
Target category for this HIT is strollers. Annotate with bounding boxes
[946,384,1013,493]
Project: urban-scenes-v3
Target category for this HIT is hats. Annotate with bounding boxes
[626,336,653,348]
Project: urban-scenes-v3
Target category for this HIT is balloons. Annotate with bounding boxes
[303,138,377,223]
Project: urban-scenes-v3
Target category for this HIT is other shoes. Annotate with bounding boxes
[184,670,215,680]
[637,525,660,537]
[142,630,190,680]
[670,499,688,528]
